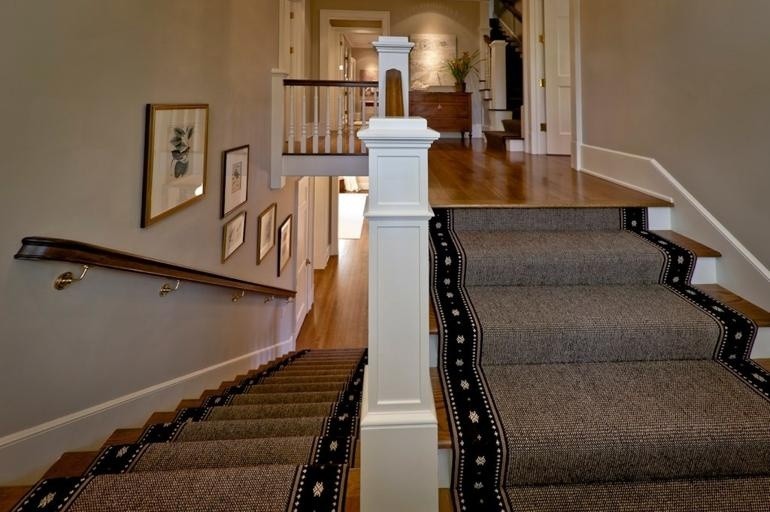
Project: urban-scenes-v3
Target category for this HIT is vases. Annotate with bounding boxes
[455,82,468,93]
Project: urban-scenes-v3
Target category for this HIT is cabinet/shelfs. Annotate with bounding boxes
[409,91,474,145]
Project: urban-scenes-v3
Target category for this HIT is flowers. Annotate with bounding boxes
[447,47,488,83]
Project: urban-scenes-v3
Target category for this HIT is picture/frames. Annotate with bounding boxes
[221,211,246,263]
[257,202,277,266]
[276,214,292,278]
[141,103,209,231]
[220,144,249,220]
